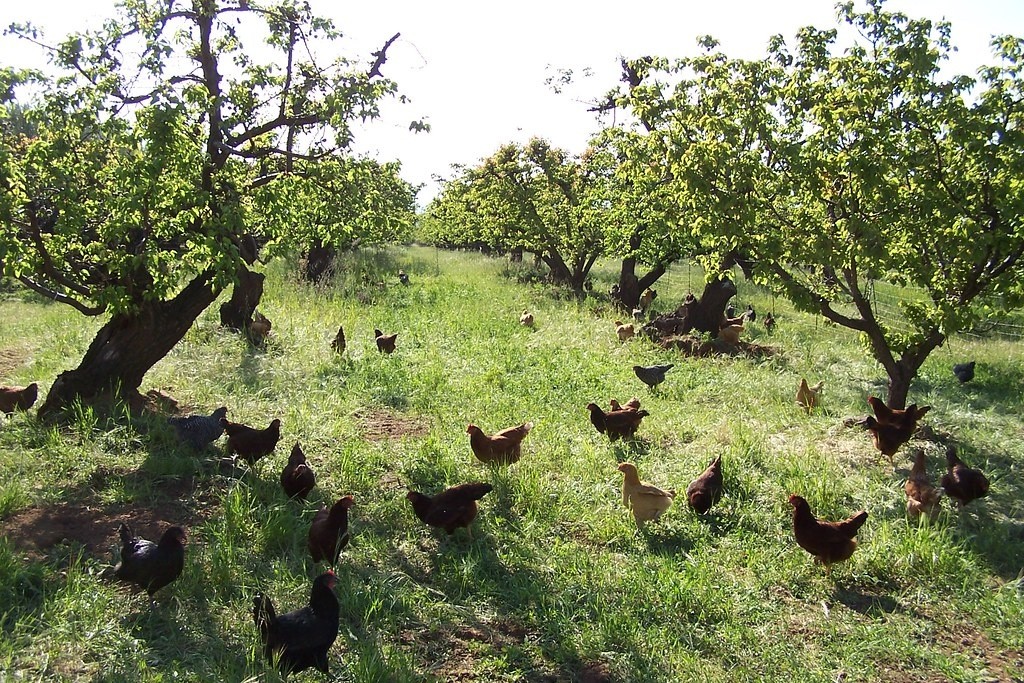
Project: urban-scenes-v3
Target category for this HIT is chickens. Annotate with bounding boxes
[0,268,1024,683]
